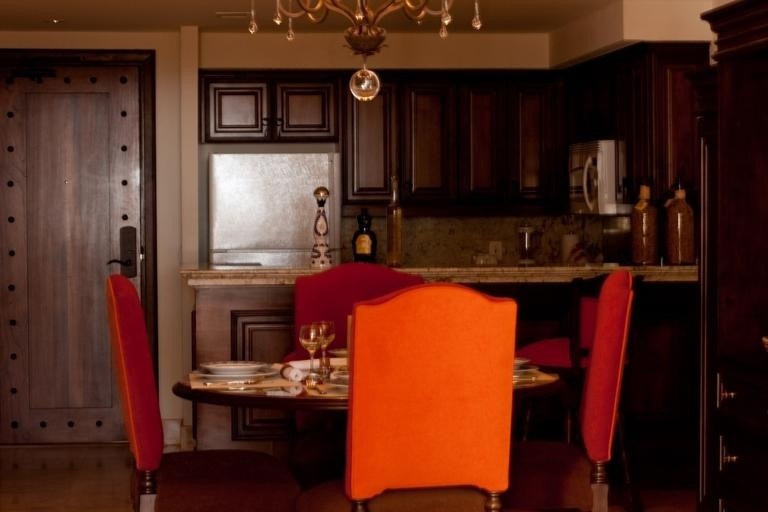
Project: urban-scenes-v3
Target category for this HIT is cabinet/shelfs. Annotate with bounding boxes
[197,40,711,216]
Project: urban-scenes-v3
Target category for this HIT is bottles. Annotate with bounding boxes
[351,175,403,267]
[512,359,539,375]
[631,172,697,264]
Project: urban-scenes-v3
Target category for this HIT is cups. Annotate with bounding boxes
[559,231,580,264]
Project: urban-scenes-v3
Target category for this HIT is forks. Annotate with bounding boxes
[305,378,327,394]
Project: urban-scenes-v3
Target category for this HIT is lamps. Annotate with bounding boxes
[242,1,484,105]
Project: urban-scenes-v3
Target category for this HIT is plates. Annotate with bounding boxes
[287,359,349,372]
[195,360,278,384]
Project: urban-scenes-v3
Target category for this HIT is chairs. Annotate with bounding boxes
[295,261,437,488]
[502,264,634,511]
[513,270,647,440]
[317,279,521,511]
[105,273,298,511]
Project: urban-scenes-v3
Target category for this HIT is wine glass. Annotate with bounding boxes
[516,223,545,268]
[299,321,336,389]
